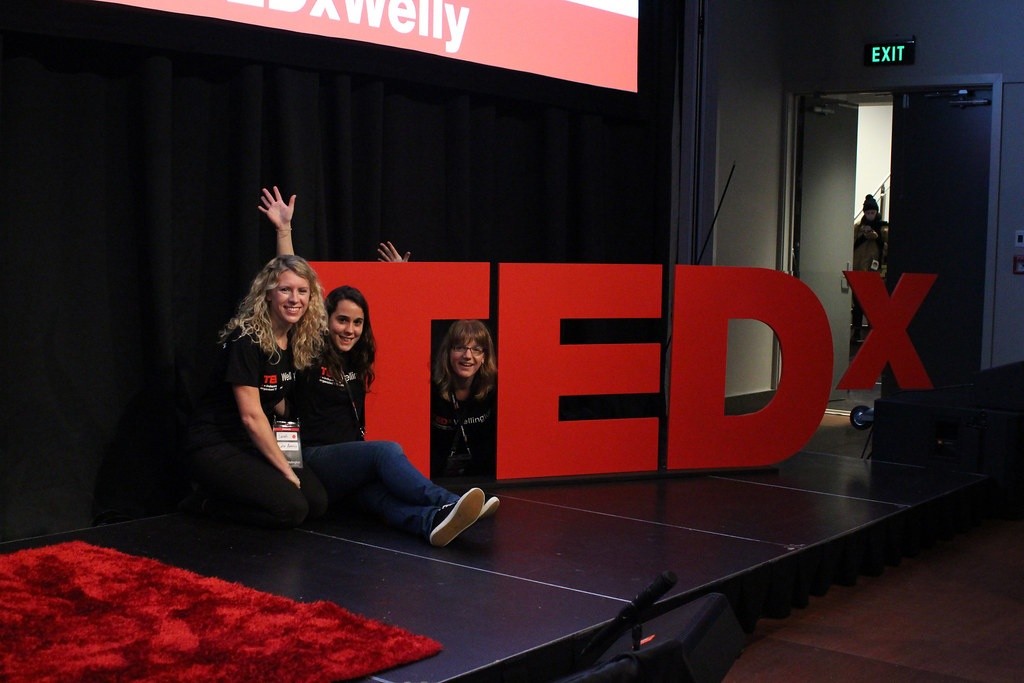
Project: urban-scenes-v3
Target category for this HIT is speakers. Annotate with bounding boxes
[590,591,748,683]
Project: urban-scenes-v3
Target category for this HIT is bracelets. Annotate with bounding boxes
[275,226,292,240]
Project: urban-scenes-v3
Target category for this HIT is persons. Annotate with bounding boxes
[173,252,337,529]
[849,195,889,343]
[369,236,498,466]
[255,182,500,549]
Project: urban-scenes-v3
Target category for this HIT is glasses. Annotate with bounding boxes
[450,345,485,355]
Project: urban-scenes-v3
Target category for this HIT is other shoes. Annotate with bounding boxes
[851,334,861,340]
[477,495,500,523]
[429,487,486,547]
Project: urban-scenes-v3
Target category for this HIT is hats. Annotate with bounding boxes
[863,195,879,211]
